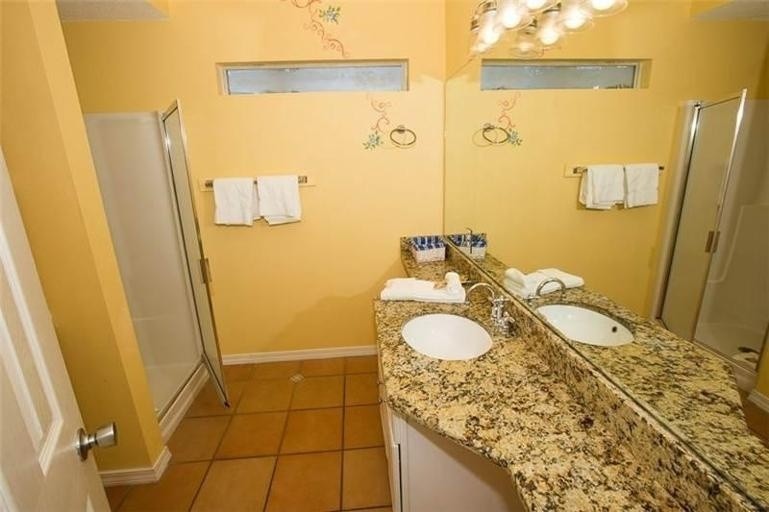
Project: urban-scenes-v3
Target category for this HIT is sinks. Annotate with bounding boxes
[531,299,636,347]
[402,309,494,360]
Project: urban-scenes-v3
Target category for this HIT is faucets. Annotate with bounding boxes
[534,276,567,302]
[465,281,496,320]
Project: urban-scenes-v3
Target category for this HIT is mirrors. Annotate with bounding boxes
[444,0,769,512]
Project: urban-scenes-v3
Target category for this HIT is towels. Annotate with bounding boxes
[579,164,625,210]
[257,175,302,226]
[381,271,467,303]
[624,163,659,208]
[213,177,261,226]
[505,266,585,300]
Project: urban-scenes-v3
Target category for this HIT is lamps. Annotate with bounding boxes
[469,0,561,44]
[517,0,629,52]
